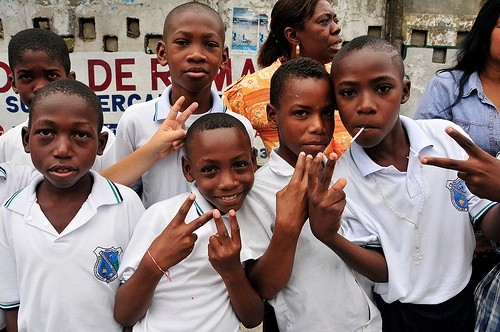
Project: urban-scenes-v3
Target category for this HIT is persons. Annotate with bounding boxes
[113,113,264,332]
[221,0,354,160]
[0,97,199,202]
[472,232,500,332]
[0,78,146,332]
[0,28,117,175]
[413,0,499,160]
[307,35,500,332]
[115,2,255,211]
[234,57,383,332]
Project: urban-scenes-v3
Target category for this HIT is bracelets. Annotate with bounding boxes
[147,250,172,283]
